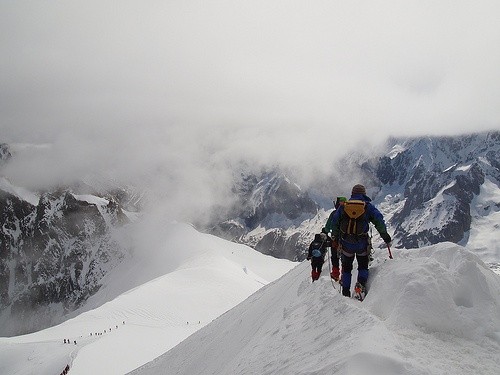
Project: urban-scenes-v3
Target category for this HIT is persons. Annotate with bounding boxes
[322,196,347,281]
[332,184,391,302]
[307,234,330,282]
[319,228,332,242]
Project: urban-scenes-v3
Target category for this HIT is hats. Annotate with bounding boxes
[351,184,366,195]
[315,234,321,240]
[336,197,347,206]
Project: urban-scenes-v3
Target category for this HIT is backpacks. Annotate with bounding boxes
[340,200,369,236]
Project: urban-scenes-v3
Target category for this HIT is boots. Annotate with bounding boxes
[339,279,343,286]
[330,266,340,281]
[312,270,320,280]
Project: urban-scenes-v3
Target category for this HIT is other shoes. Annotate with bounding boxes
[355,283,366,301]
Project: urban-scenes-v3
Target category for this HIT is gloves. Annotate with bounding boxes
[382,235,391,243]
[321,228,328,238]
